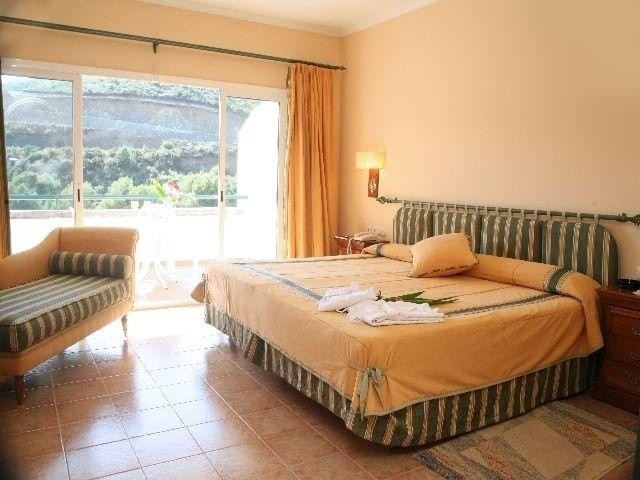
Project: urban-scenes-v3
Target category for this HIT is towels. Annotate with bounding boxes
[318,282,445,327]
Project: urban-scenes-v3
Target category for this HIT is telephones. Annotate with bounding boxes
[354,232,378,241]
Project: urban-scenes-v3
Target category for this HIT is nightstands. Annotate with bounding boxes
[333,235,390,254]
[595,284,640,416]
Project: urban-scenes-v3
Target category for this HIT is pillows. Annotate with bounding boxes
[48,251,133,279]
[410,231,479,279]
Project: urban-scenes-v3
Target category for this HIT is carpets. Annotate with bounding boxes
[411,398,638,480]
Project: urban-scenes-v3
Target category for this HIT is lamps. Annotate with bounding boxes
[356,151,384,169]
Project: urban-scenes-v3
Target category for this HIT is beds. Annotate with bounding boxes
[190,207,616,447]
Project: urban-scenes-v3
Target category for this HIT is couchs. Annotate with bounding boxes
[2,225,139,404]
